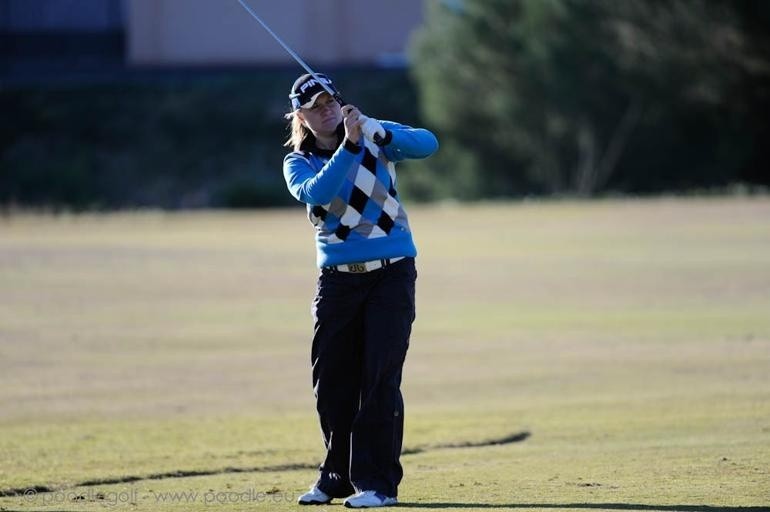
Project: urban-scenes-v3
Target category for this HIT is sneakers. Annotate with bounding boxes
[342,487,401,508]
[297,482,336,505]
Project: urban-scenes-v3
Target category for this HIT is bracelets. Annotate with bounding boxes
[341,136,362,155]
[373,129,394,148]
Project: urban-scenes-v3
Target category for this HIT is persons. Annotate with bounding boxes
[281,71,440,507]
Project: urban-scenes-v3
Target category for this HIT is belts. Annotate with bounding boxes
[323,255,407,274]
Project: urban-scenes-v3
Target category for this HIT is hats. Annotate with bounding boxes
[288,71,342,111]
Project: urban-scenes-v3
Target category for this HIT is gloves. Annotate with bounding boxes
[358,111,387,145]
